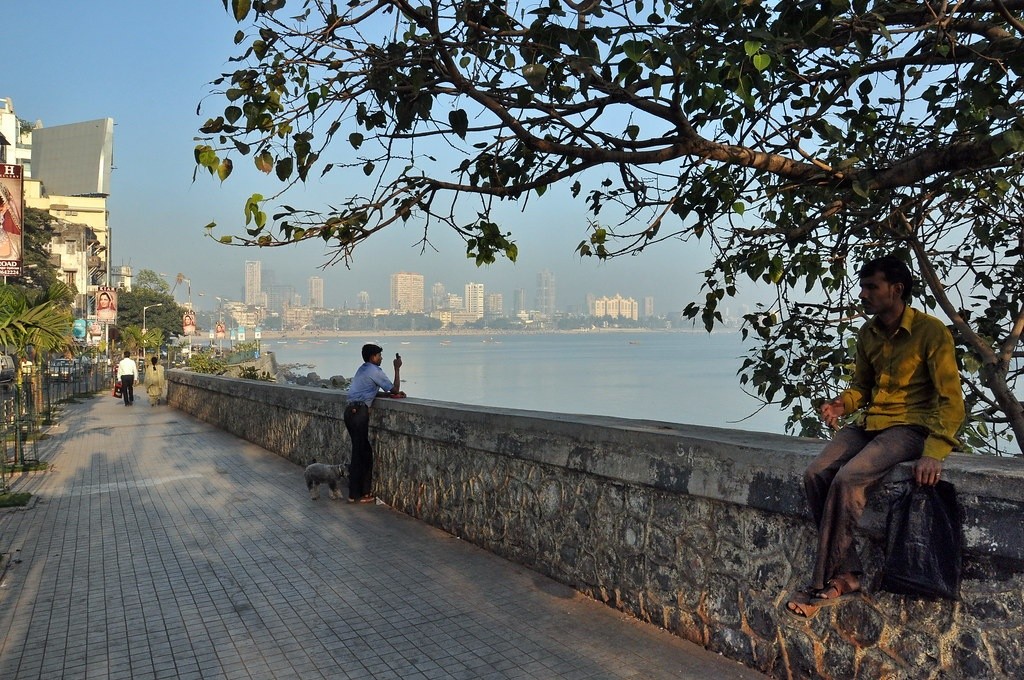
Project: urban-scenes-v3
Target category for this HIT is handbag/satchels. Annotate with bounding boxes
[112,383,122,398]
[870,480,964,601]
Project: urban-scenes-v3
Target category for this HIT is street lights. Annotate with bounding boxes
[142,304,162,368]
[158,273,192,359]
[199,294,262,358]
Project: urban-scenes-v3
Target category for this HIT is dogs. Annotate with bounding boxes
[304,463,350,500]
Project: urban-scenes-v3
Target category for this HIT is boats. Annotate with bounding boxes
[401,341,410,345]
[295,338,329,345]
[440,339,452,345]
[277,340,287,344]
[481,337,502,344]
[338,340,348,344]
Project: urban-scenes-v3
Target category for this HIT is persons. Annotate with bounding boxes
[216,325,225,337]
[145,357,164,406]
[0,182,21,260]
[117,351,137,406]
[183,315,195,332]
[97,292,116,319]
[786,257,966,622]
[344,344,402,503]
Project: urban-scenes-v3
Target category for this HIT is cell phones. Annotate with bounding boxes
[396,353,399,360]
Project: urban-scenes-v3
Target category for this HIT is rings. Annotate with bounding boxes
[936,474,940,476]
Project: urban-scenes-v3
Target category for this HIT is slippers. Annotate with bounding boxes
[784,587,823,622]
[809,577,863,607]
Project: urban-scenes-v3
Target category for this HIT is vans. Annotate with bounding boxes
[1,356,16,393]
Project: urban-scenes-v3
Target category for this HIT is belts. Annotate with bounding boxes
[347,401,366,408]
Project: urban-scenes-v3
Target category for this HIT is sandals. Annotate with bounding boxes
[360,492,377,497]
[348,496,375,504]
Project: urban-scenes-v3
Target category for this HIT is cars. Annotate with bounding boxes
[48,359,84,382]
